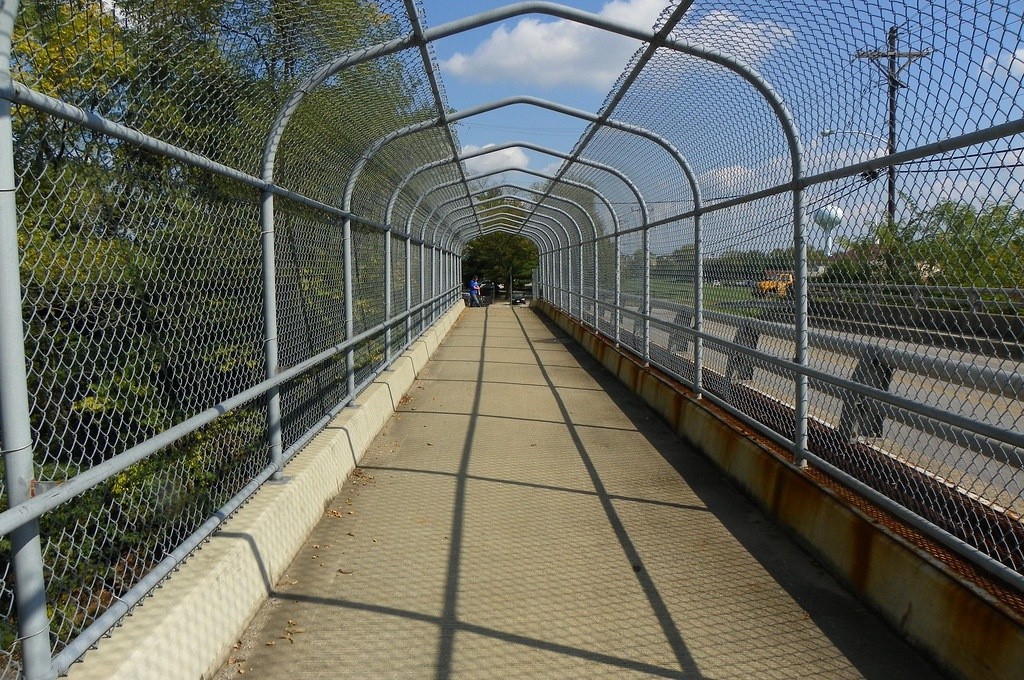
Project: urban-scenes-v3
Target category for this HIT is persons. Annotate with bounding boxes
[469,275,482,307]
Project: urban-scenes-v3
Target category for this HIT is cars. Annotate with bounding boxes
[511,294,527,304]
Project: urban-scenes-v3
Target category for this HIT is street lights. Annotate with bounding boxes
[821,129,897,284]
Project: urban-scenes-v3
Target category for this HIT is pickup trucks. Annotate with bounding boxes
[755,273,829,298]
